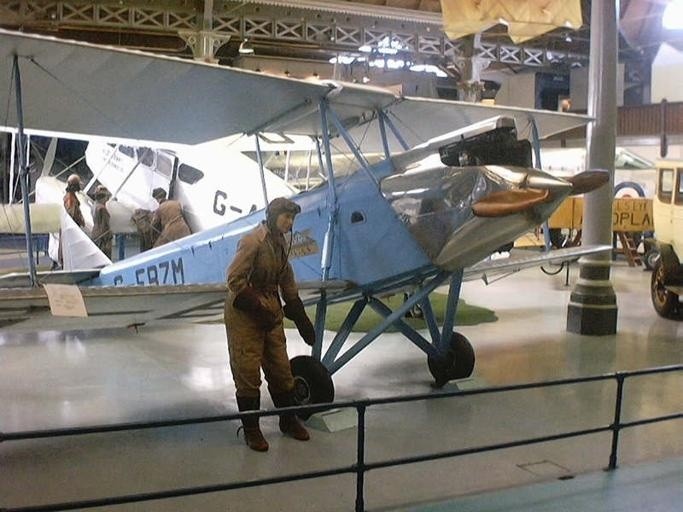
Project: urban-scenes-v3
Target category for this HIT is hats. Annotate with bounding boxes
[152,187,166,199]
[94,185,112,196]
[68,174,84,185]
[266,197,301,230]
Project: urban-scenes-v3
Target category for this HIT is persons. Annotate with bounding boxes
[221,197,314,451]
[148,187,190,248]
[89,185,112,260]
[62,174,86,227]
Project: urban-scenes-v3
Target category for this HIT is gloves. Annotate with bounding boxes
[283,298,316,346]
[233,288,271,312]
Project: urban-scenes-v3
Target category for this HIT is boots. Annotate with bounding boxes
[267,384,310,442]
[235,388,269,452]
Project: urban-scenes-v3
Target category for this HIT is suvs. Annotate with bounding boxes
[651,160,683,319]
